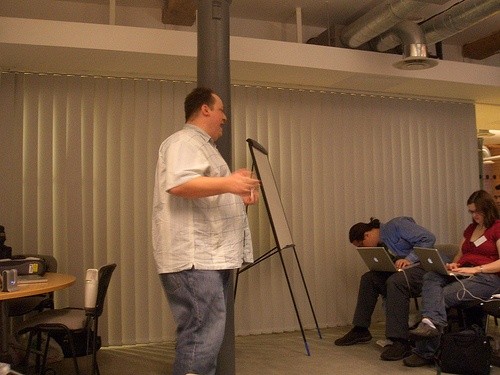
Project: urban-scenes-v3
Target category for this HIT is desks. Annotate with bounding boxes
[0,271,76,362]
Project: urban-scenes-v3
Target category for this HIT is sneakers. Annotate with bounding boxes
[380,343,411,360]
[335,328,372,346]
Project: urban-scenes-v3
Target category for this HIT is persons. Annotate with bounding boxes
[401,190,500,367]
[494,184,500,208]
[151,88,261,375]
[335,215,436,362]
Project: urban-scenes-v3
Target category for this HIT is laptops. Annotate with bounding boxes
[355,247,420,273]
[413,247,474,276]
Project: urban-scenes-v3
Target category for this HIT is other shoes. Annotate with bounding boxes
[403,354,431,367]
[409,323,440,337]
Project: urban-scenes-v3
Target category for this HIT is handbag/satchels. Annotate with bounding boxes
[440,336,490,375]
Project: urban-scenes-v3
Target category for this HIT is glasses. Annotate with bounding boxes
[468,209,478,214]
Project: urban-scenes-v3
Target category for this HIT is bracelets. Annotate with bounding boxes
[480,265,483,273]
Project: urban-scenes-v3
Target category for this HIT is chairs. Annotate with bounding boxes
[9,254,57,318]
[457,297,500,353]
[15,263,117,375]
[409,243,458,311]
